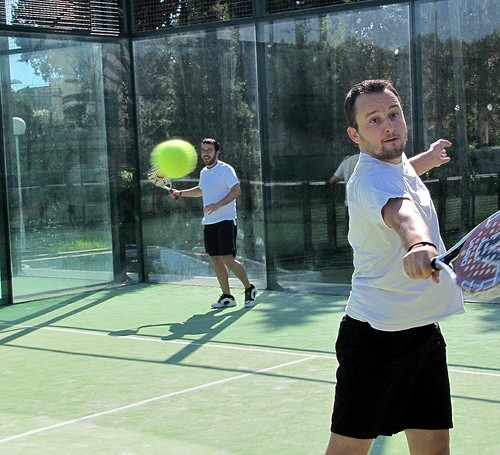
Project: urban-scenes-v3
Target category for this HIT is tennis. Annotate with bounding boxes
[151,139,199,180]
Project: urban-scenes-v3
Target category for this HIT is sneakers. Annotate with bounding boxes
[211,294,236,308]
[244,284,258,308]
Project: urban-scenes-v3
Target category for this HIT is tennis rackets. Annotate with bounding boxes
[147,168,180,200]
[429,211,500,295]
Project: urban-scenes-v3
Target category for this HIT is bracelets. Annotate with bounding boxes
[407,241,437,251]
[179,190,182,196]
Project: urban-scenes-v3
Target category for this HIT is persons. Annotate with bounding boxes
[330,154,359,234]
[171,139,259,308]
[317,80,456,455]
